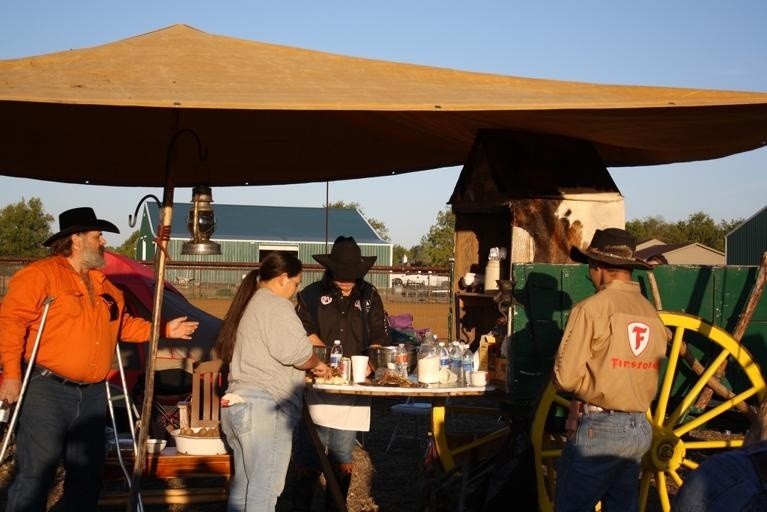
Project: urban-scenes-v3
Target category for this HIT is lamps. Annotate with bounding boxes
[180,161,221,255]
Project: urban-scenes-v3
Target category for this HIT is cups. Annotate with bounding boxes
[350,355,369,384]
[439,368,458,385]
[465,371,488,386]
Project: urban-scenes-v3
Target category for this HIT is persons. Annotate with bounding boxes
[216,252,317,512]
[2,208,199,512]
[278,236,388,512]
[553,229,671,511]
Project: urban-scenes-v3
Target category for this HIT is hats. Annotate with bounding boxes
[40,206,121,247]
[311,235,378,281]
[568,227,654,271]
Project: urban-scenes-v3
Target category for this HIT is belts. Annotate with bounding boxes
[578,403,626,413]
[32,360,106,388]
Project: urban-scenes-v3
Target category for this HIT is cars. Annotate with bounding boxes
[393,271,451,290]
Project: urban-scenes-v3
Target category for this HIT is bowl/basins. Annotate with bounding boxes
[170,427,232,457]
[144,438,167,454]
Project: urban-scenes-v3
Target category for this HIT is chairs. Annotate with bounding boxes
[103,381,149,451]
[382,396,432,463]
[176,359,231,429]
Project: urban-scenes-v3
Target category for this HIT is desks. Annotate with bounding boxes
[96,446,233,511]
[305,383,506,510]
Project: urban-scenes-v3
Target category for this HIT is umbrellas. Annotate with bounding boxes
[2,23,766,509]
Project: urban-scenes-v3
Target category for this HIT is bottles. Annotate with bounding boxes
[388,363,395,385]
[398,343,407,382]
[463,344,474,384]
[439,342,450,377]
[418,331,441,385]
[330,340,341,368]
[452,341,461,377]
[342,358,350,384]
